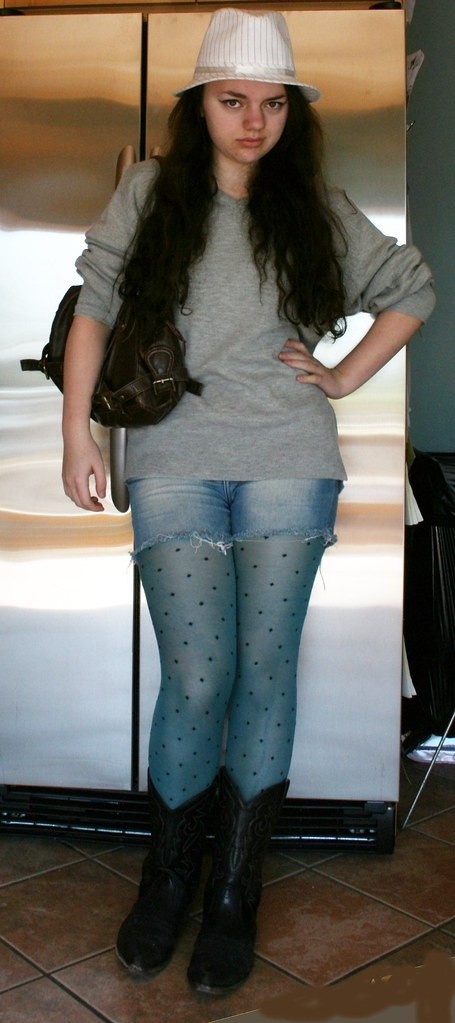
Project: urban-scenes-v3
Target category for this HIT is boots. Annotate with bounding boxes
[188,770,290,997]
[115,766,225,979]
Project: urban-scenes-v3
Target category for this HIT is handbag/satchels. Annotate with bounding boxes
[40,285,204,426]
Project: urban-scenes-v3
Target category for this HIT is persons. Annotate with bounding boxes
[62,7,436,996]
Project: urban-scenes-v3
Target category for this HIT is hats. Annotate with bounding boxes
[172,6,322,102]
